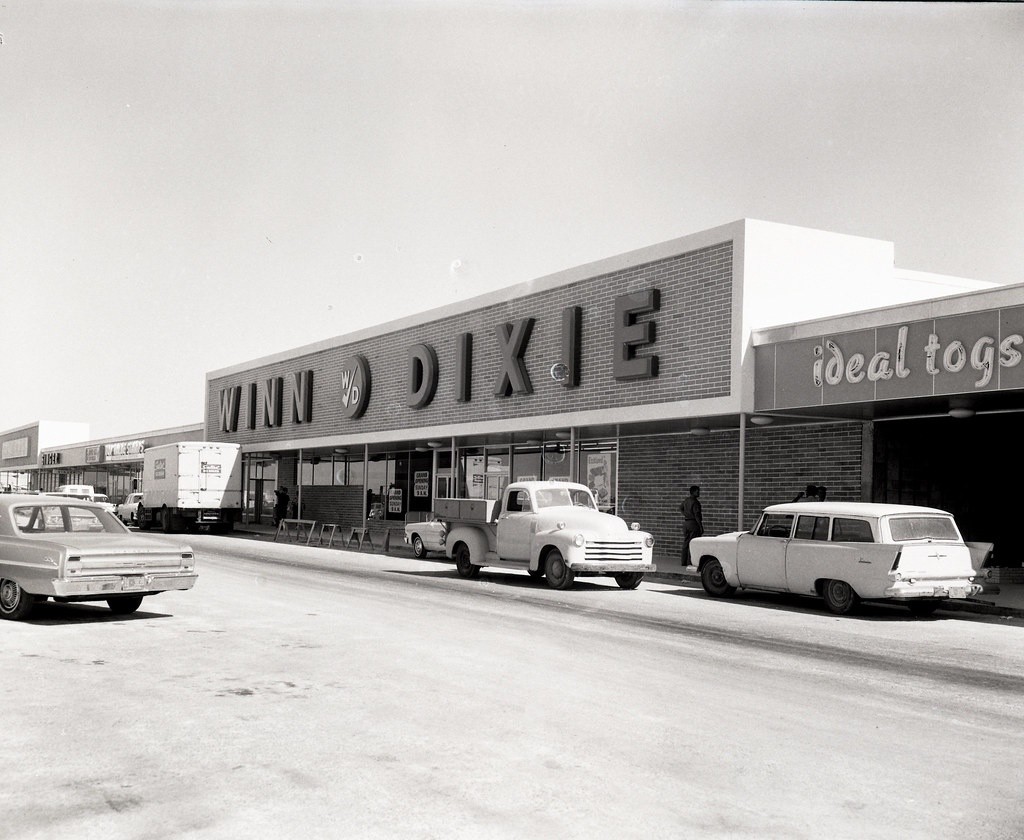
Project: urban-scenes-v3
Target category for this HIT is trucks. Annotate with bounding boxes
[137,442,243,536]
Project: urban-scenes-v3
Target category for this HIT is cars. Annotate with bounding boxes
[0,494,198,621]
[404,514,449,559]
[686,502,992,616]
[369,503,383,519]
[39,485,118,515]
[118,492,144,526]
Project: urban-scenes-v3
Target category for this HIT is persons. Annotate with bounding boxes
[791,485,818,503]
[816,486,830,502]
[273,487,290,531]
[290,491,306,529]
[679,486,704,567]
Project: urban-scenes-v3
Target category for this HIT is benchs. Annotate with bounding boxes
[345,526,374,551]
[276,519,322,545]
[316,523,344,548]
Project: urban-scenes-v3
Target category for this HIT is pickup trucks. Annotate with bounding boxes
[433,480,658,590]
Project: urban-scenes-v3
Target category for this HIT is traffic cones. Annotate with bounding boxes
[382,527,391,553]
